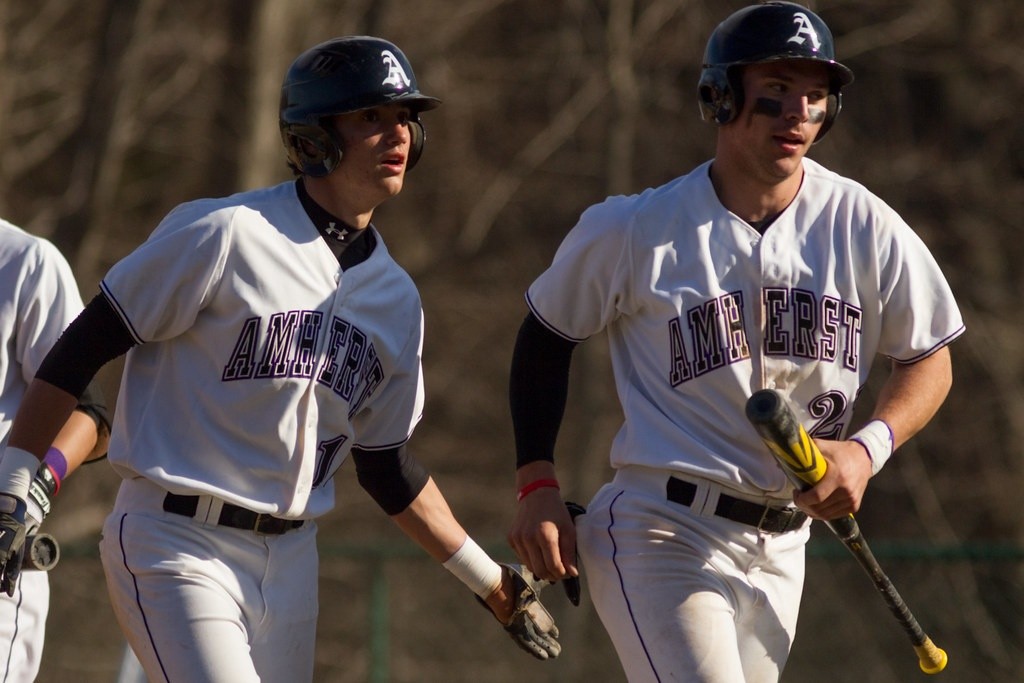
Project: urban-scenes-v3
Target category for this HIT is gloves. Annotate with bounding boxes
[0,492,27,597]
[472,562,561,662]
[25,462,60,557]
[532,502,587,608]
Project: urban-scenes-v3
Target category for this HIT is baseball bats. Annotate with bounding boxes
[744,387,951,674]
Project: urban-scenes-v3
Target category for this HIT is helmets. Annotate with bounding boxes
[696,1,854,145]
[278,36,443,177]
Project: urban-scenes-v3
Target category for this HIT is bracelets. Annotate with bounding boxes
[517,476,561,501]
[442,534,503,599]
[0,446,41,502]
[847,417,895,475]
[37,446,68,497]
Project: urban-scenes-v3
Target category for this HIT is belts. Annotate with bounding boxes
[163,490,305,537]
[666,477,808,535]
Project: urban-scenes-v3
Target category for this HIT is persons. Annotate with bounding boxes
[1,36,560,683]
[510,3,964,683]
[0,215,114,683]
